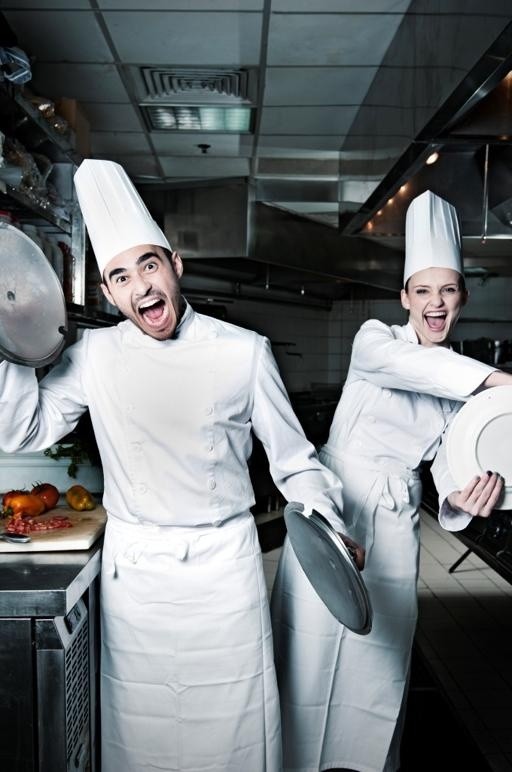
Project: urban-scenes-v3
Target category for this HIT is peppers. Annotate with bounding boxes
[66,485,96,510]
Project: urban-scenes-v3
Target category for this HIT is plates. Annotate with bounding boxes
[445,386,512,511]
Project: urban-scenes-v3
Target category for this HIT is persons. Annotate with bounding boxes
[0,243,366,772]
[270,266,512,771]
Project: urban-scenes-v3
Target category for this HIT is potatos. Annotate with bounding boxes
[9,495,46,515]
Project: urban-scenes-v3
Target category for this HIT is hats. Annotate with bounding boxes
[403,190,466,290]
[73,159,173,283]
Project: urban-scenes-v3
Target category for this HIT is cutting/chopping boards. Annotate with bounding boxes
[1,503,107,553]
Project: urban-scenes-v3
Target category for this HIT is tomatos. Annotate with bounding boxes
[3,489,29,505]
[30,480,59,508]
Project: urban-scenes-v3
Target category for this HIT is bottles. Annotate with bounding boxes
[23,223,64,285]
